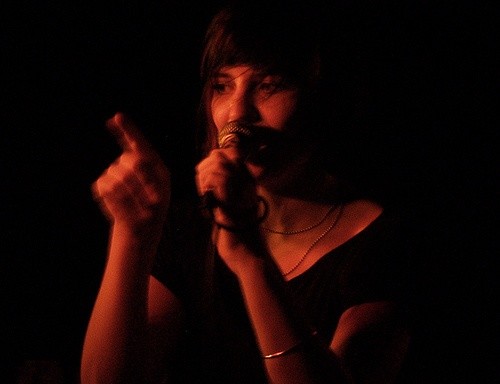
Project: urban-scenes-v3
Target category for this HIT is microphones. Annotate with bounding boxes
[204,120,256,211]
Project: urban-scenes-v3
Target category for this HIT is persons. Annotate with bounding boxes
[79,4,400,383]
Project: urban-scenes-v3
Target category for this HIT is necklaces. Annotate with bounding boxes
[280,200,345,280]
[253,189,336,235]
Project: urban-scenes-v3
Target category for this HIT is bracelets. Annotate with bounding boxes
[258,322,308,359]
